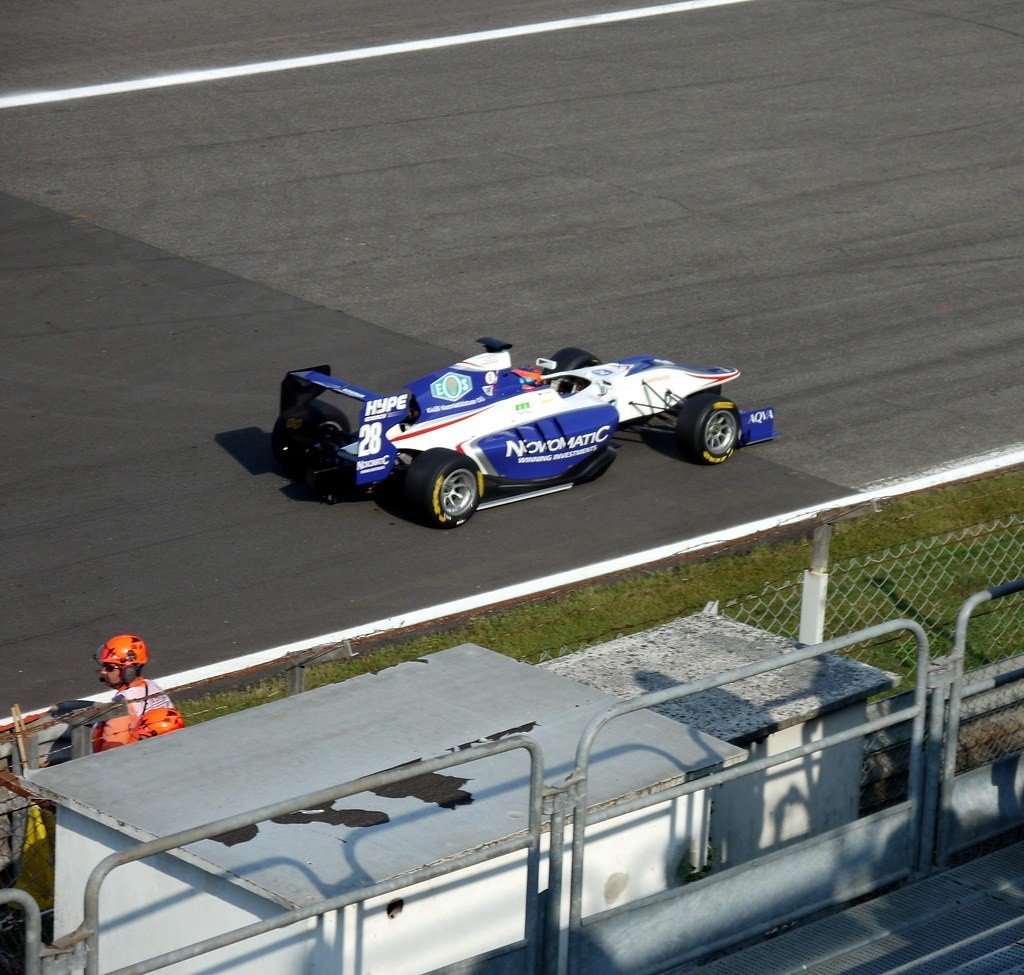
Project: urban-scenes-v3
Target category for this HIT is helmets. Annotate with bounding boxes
[509,364,544,392]
[93,633,148,670]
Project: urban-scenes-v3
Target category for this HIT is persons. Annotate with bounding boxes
[513,364,542,389]
[92,634,184,752]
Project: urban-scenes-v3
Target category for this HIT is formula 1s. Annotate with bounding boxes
[270,333,776,530]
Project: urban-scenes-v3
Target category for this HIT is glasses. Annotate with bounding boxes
[515,376,537,387]
[102,662,119,672]
